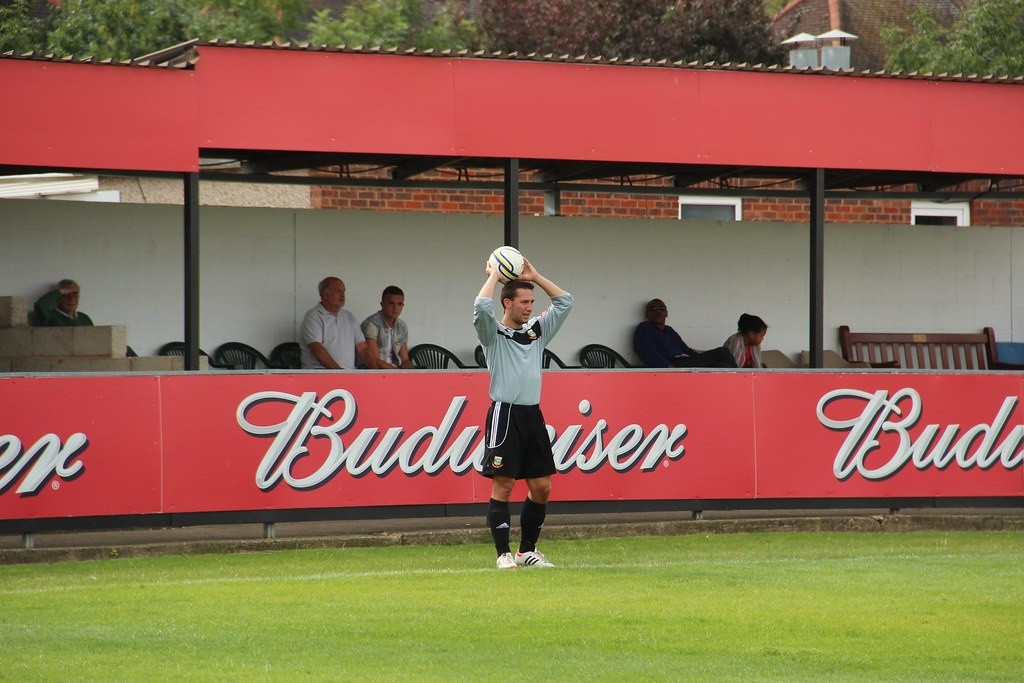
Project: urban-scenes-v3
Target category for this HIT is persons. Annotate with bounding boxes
[301,277,395,370]
[634,299,738,368]
[474,257,575,568]
[724,313,767,367]
[356,286,414,369]
[35,279,138,357]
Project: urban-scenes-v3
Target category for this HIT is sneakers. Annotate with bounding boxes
[515,546,555,568]
[497,552,517,569]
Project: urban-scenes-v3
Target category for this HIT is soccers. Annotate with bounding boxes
[489,245,525,284]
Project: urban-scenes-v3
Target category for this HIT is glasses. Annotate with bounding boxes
[648,306,667,312]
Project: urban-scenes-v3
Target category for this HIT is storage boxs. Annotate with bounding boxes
[0,296,208,373]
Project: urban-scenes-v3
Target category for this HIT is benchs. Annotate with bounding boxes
[839,324,1024,371]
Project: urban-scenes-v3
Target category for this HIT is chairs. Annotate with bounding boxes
[215,343,273,369]
[800,350,871,368]
[270,342,302,370]
[474,345,586,369]
[408,344,480,369]
[579,344,638,368]
[159,342,235,370]
[761,350,799,369]
